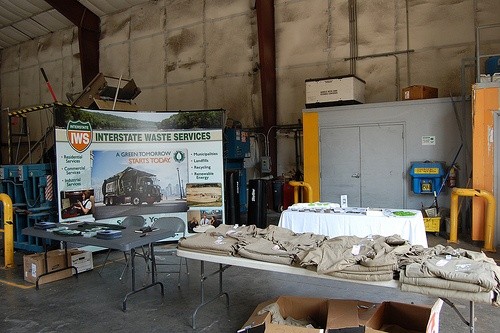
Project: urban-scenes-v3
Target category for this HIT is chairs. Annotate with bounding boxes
[98,215,190,281]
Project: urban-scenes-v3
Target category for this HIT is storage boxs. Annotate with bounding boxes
[24,250,94,284]
[424,217,441,232]
[305,74,366,107]
[237,295,444,333]
[401,84,438,101]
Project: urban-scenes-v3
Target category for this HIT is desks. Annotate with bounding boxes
[280,206,428,248]
[21,222,175,312]
[176,248,476,333]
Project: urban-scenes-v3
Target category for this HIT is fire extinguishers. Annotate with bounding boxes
[448,161,457,188]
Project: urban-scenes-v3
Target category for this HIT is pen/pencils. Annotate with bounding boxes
[140,233,146,237]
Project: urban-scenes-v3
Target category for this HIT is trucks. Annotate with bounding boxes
[101,167,161,206]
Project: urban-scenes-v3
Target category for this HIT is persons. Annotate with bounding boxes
[200,214,210,225]
[211,216,219,228]
[190,217,199,232]
[73,191,93,216]
